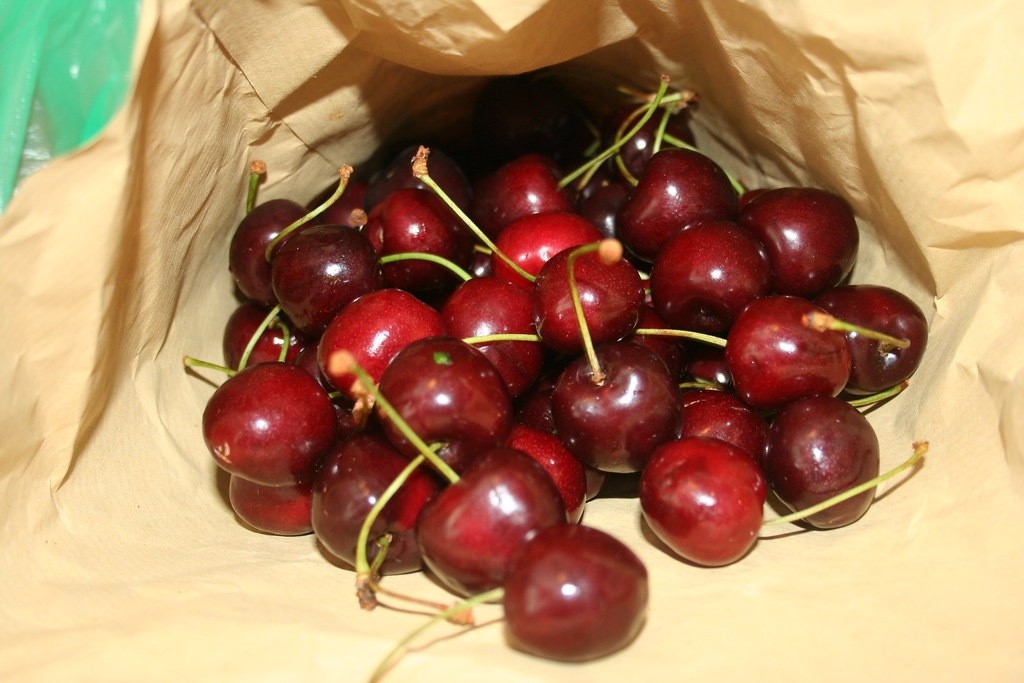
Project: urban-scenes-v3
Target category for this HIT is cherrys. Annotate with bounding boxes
[181,73,928,683]
